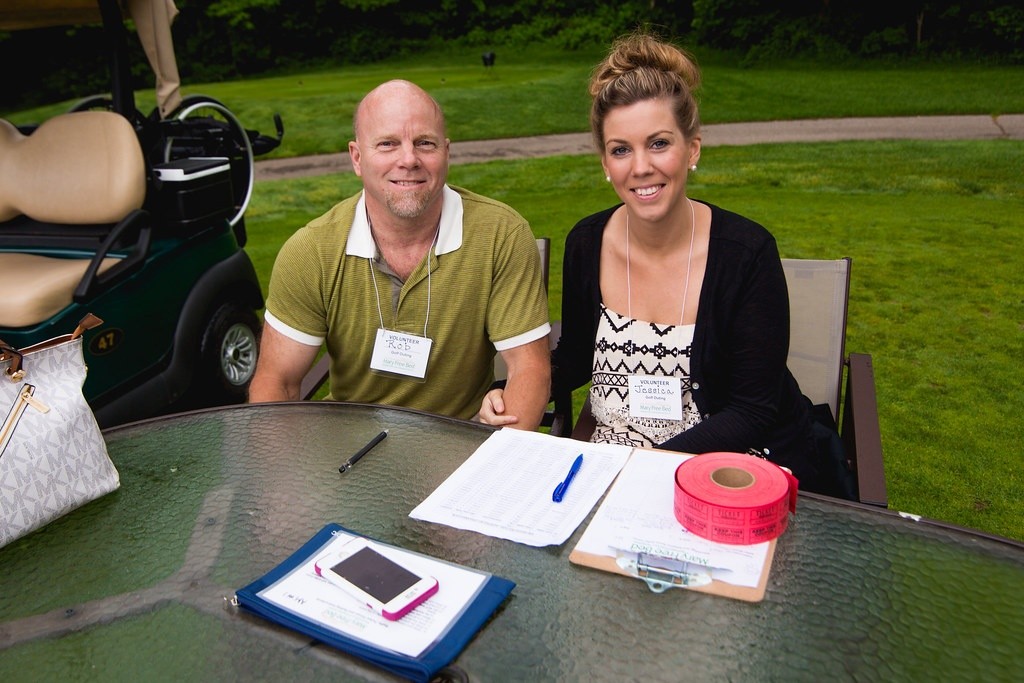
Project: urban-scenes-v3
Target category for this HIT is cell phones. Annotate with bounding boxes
[316,536,439,621]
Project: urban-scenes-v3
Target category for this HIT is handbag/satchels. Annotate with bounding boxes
[0,313,122,551]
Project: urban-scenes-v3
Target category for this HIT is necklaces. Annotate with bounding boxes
[249,79,552,435]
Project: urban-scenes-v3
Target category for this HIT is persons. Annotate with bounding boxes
[477,32,861,502]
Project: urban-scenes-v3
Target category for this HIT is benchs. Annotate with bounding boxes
[0,107,147,334]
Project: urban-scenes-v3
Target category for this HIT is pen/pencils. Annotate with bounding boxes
[339,429,390,474]
[552,454,583,502]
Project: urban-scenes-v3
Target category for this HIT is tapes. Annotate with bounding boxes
[674,450,800,545]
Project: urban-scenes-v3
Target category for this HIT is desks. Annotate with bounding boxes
[0,403,1023,683]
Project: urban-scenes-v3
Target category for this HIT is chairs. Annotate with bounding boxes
[300,238,889,513]
